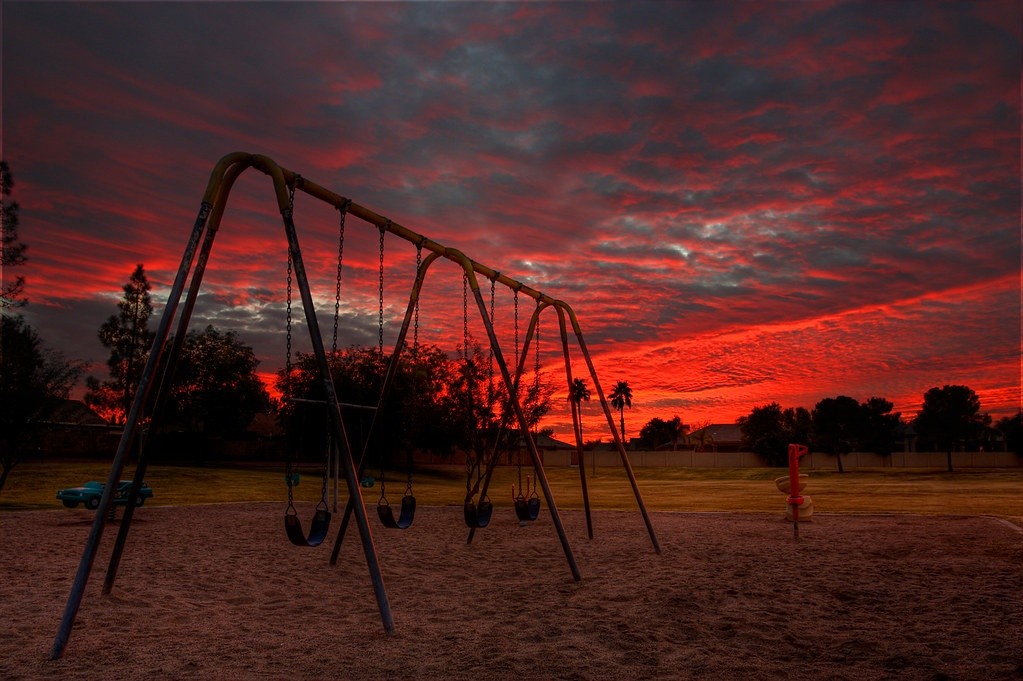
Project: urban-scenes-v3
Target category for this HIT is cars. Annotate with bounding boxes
[56,480,154,511]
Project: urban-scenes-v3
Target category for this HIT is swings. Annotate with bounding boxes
[463,272,494,527]
[376,228,417,529]
[513,290,541,521]
[285,188,344,548]
